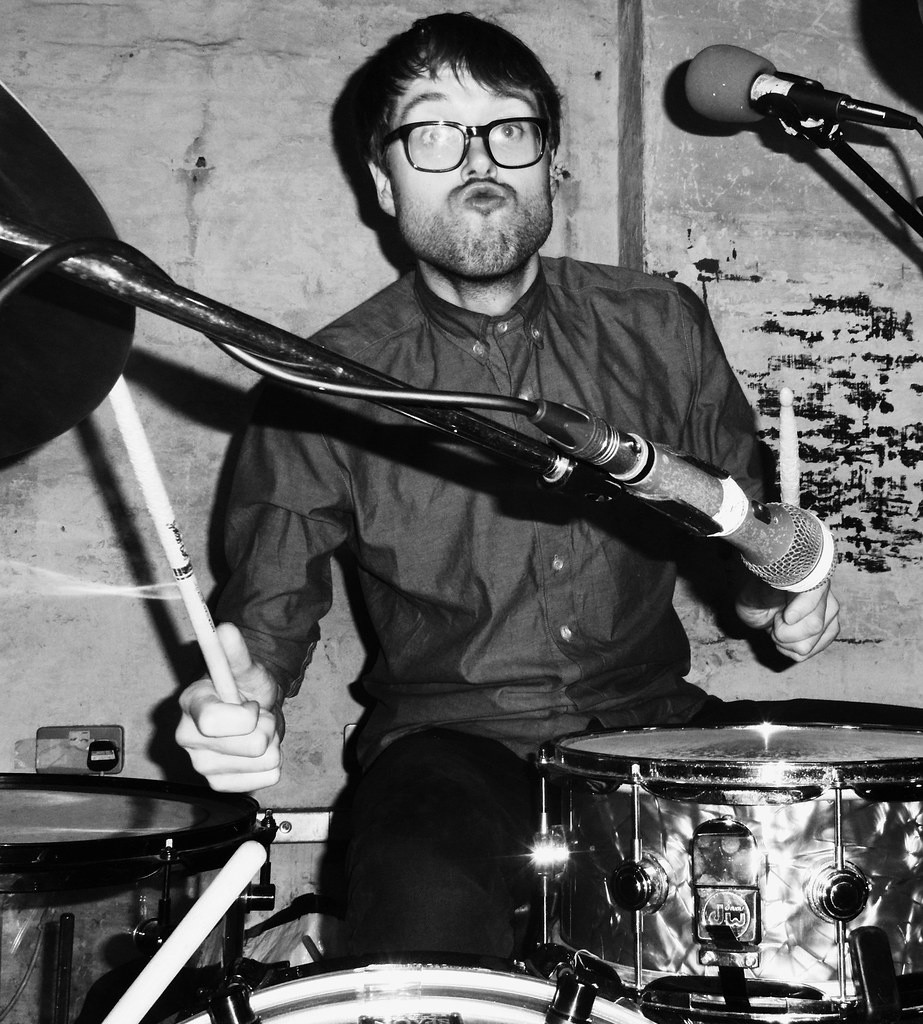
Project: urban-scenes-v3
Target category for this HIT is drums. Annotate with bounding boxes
[169,964,655,1024]
[537,717,923,1023]
[1,773,260,1024]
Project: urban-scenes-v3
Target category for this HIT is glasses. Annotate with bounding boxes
[378,117,549,172]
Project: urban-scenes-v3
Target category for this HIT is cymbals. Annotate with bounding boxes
[0,83,136,473]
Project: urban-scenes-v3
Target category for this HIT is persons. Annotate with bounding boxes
[172,12,839,970]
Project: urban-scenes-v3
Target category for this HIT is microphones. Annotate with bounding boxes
[686,43,917,131]
[529,400,839,593]
